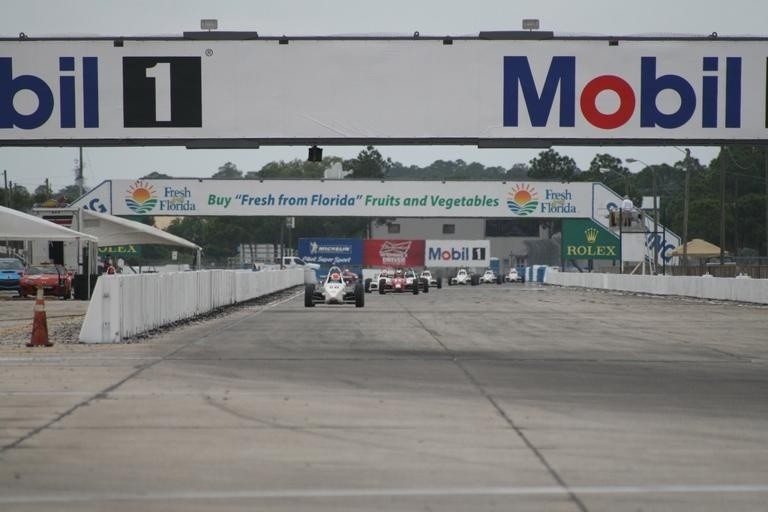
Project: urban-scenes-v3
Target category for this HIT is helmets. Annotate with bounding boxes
[331,273,340,281]
[381,270,403,276]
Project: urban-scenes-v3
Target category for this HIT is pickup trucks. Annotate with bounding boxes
[256,256,321,271]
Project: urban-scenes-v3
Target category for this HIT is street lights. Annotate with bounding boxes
[627,156,658,275]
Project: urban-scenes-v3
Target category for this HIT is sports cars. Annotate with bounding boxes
[447,266,526,286]
[304,266,365,307]
[365,268,442,295]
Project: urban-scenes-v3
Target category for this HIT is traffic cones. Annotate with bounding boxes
[26,286,53,347]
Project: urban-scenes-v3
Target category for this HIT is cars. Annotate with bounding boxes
[0,258,71,298]
